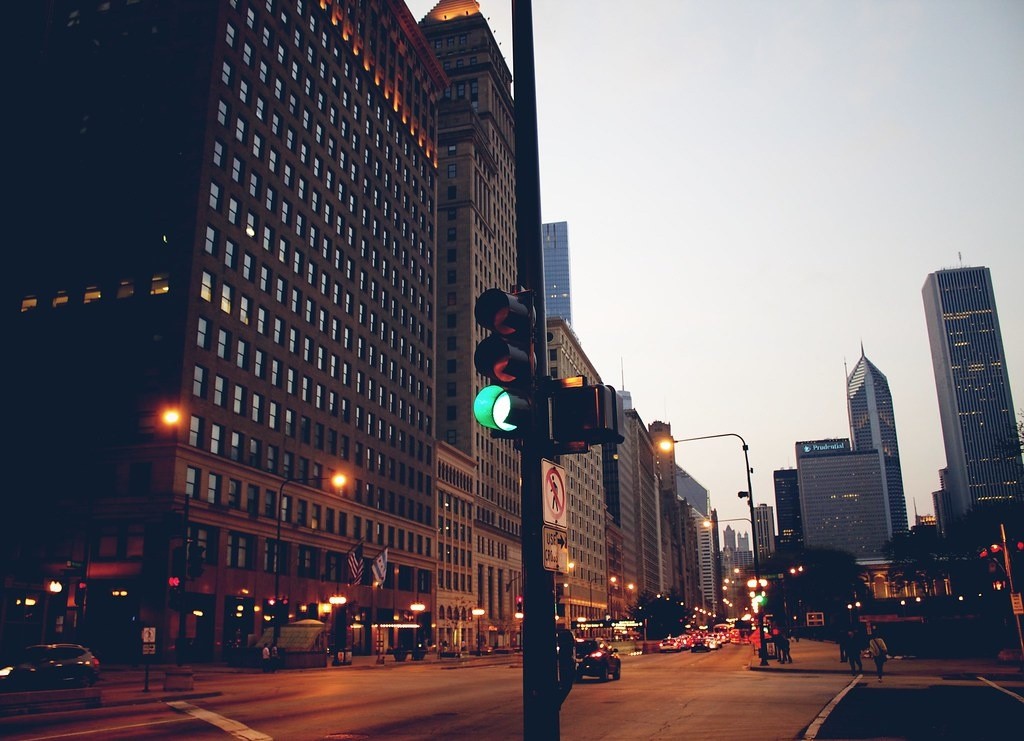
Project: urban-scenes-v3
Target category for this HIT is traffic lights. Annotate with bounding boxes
[168,569,183,596]
[279,597,291,623]
[517,596,524,614]
[188,544,206,578]
[475,287,550,441]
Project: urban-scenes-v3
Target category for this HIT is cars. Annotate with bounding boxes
[659,622,749,657]
[570,637,625,681]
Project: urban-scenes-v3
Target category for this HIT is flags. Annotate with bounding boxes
[347,543,364,587]
[372,547,387,590]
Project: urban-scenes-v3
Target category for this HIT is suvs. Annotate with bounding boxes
[0,643,101,688]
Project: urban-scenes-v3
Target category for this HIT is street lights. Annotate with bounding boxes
[472,609,487,656]
[661,433,770,668]
[269,474,345,649]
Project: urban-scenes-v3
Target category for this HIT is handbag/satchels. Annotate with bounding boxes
[880,650,888,660]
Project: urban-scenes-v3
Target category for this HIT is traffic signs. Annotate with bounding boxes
[541,525,569,573]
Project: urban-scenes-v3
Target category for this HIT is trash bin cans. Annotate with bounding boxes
[343,648,353,665]
[332,649,344,665]
[765,638,777,659]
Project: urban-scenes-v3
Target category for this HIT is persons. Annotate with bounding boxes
[869,634,887,683]
[262,643,270,672]
[834,624,867,675]
[771,631,792,664]
